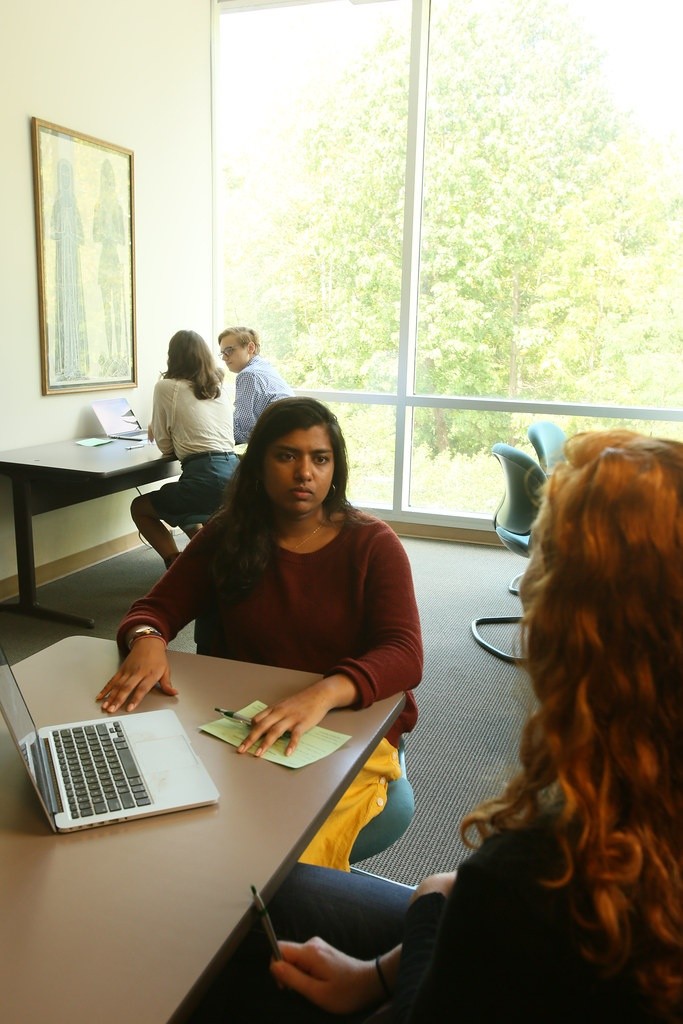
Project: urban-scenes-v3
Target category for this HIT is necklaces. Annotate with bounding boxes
[280,518,325,550]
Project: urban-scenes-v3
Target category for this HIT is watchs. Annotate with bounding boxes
[128,626,161,649]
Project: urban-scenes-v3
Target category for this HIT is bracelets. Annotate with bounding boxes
[376,955,388,994]
[130,635,166,649]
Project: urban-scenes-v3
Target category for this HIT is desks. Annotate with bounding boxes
[0,636,405,1024]
[0,431,178,629]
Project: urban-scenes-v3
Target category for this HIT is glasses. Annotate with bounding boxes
[218,342,250,359]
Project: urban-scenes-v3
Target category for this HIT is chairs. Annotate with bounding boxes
[468,419,571,664]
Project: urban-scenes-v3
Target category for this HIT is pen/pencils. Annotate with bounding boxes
[250,885,282,960]
[126,445,144,449]
[214,706,291,738]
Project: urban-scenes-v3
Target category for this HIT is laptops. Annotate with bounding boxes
[90,398,149,441]
[0,644,220,834]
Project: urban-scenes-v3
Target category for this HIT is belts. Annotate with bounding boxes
[181,452,234,466]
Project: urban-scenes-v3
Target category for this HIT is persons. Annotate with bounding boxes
[130,330,239,571]
[217,327,296,461]
[96,397,423,874]
[187,427,683,1024]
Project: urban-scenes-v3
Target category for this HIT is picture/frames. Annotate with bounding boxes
[31,116,138,396]
